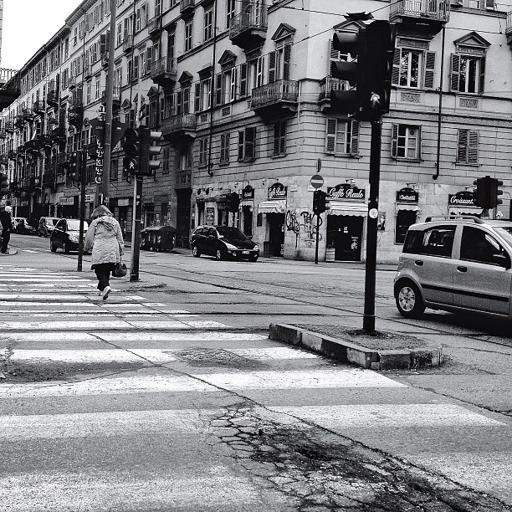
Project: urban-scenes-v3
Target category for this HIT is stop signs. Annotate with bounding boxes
[310,175,323,188]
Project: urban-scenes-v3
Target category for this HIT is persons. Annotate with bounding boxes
[82,204,126,301]
[1,205,15,254]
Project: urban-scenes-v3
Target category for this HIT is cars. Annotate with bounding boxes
[0,217,92,255]
[189,225,259,261]
[394,215,511,322]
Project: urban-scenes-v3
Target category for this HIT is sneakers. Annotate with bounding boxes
[96,285,112,300]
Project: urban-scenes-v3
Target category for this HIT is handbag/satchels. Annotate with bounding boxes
[110,260,128,278]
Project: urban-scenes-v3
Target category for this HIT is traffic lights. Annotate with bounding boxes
[67,152,81,182]
[123,125,162,176]
[313,190,329,214]
[472,176,502,208]
[330,20,395,121]
